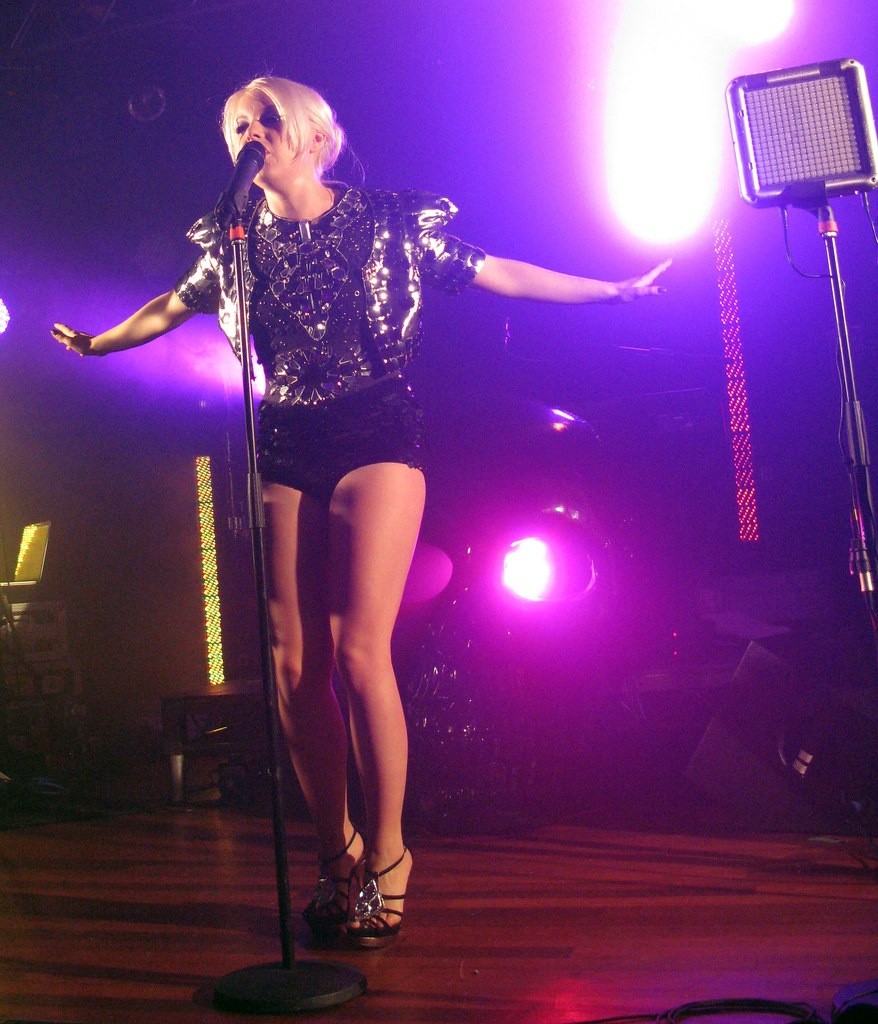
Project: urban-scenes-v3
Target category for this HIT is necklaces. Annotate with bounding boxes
[265,188,332,244]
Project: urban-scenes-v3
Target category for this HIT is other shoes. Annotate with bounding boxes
[350,845,407,947]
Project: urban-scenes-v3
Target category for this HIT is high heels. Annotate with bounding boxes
[302,825,365,925]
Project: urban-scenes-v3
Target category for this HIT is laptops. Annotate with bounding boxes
[0,521,52,587]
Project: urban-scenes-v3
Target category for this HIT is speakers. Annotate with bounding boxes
[686,641,878,835]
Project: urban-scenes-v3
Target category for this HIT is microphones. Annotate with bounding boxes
[214,141,266,231]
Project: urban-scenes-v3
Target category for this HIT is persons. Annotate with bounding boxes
[53,78,674,952]
